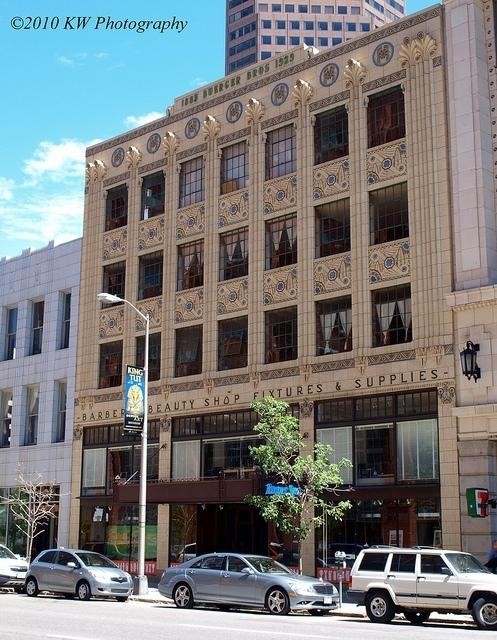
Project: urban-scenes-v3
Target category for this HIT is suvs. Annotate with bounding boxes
[0,541,28,593]
[344,543,496,632]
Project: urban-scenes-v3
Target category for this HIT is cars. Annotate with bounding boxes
[176,543,196,561]
[268,538,367,568]
[155,552,341,615]
[21,544,134,604]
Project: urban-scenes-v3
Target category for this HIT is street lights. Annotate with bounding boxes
[92,291,150,596]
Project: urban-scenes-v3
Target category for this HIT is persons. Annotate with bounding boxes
[487,540,497,561]
